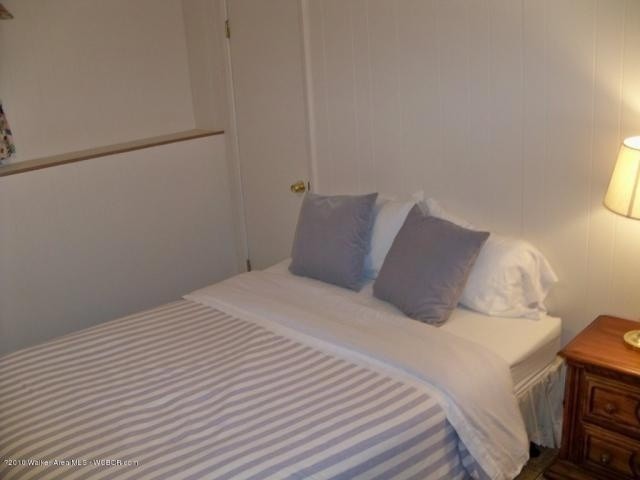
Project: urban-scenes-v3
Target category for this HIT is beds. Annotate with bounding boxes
[1,255,564,480]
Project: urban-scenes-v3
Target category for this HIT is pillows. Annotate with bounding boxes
[288,189,558,327]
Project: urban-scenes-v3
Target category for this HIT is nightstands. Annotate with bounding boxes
[542,313,640,480]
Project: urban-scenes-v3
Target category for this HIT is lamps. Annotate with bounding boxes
[603,136,640,350]
